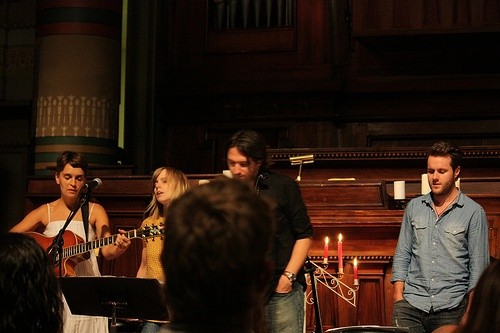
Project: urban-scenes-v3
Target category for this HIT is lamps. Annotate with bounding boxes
[289,154,315,179]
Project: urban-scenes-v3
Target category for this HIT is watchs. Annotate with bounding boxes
[282,271,296,282]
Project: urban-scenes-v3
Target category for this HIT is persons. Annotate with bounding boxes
[226,128,314,333]
[390,141,490,333]
[135,166,190,333]
[431,258,500,333]
[9,151,131,333]
[0,231,63,333]
[158,177,282,333]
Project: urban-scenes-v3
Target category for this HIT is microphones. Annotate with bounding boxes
[83,177,103,188]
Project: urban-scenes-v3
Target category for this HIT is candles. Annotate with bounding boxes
[337,233,344,273]
[455,177,461,190]
[323,236,330,263]
[421,174,432,195]
[394,181,405,200]
[353,257,359,284]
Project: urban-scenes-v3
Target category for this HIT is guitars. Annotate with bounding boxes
[20,222,166,278]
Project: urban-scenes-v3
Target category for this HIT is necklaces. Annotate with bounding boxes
[434,196,457,218]
